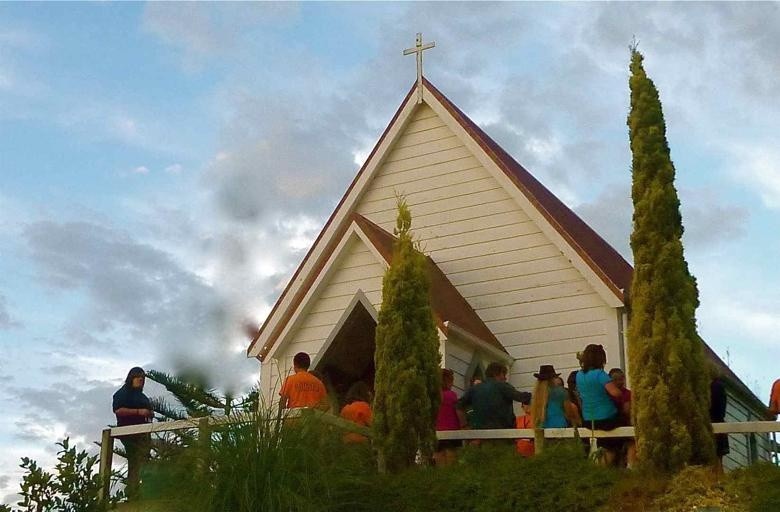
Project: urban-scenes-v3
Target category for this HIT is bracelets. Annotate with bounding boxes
[460,423,467,427]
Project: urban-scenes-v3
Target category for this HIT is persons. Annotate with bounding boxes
[112,367,156,497]
[309,369,322,383]
[469,375,482,386]
[576,343,627,468]
[432,368,466,467]
[708,374,730,476]
[608,367,635,469]
[454,361,533,447]
[278,352,332,427]
[514,401,535,457]
[530,364,574,427]
[565,350,583,426]
[557,377,565,388]
[338,380,375,445]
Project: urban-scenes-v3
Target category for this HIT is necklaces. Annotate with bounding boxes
[524,415,530,429]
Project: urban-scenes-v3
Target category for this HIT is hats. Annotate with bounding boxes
[533,365,561,379]
